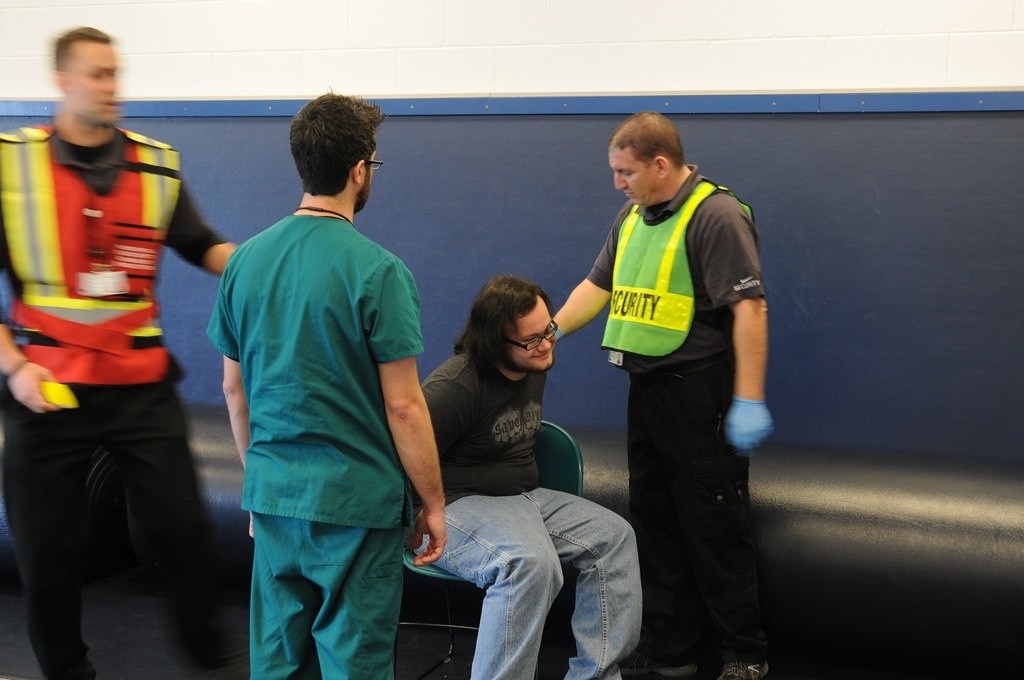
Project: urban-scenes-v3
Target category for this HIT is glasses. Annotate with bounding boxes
[504,319,558,350]
[347,158,383,169]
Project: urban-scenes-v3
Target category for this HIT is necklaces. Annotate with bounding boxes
[295,207,351,223]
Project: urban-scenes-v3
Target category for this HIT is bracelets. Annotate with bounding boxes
[550,323,561,341]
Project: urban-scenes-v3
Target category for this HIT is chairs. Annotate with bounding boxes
[394,419,583,680]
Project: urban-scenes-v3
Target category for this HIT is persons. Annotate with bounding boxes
[208,98,446,679]
[0,29,243,680]
[552,110,776,680]
[395,272,642,680]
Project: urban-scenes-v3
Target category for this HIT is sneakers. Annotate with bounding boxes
[618,649,698,676]
[716,654,768,680]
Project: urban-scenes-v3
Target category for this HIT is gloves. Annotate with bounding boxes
[725,396,773,450]
[556,328,563,342]
